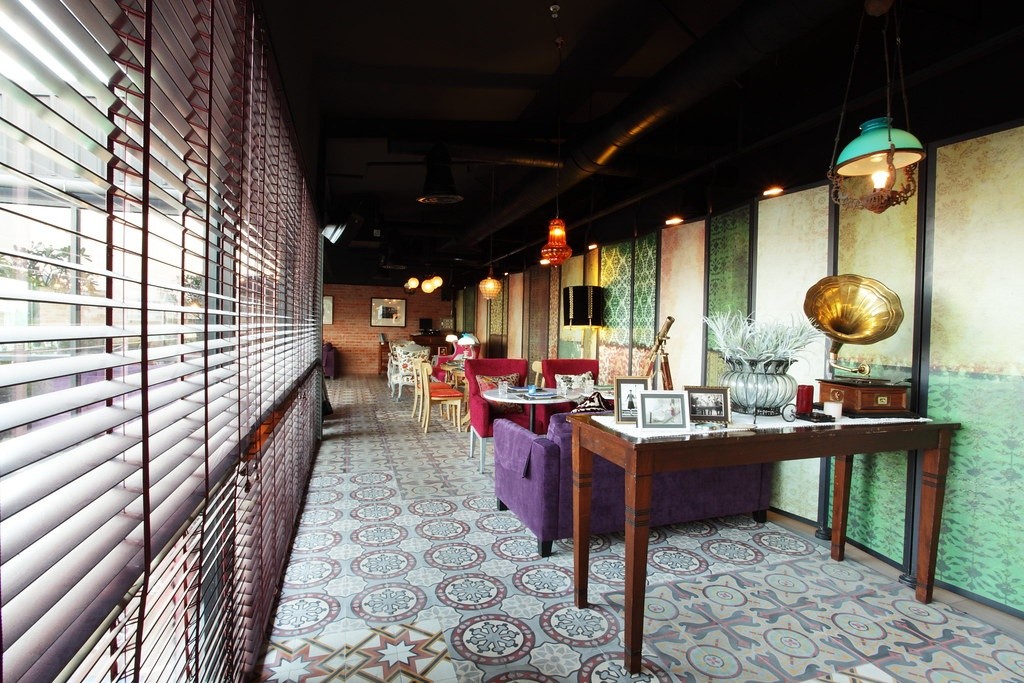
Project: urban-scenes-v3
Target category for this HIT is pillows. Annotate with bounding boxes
[554,371,594,404]
[570,391,613,413]
[475,372,524,414]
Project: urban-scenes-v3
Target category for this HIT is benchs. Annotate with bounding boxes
[494,413,773,558]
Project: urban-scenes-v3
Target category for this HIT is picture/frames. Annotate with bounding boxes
[438,347,447,356]
[370,297,406,328]
[613,375,731,430]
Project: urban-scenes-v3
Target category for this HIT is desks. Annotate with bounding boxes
[451,368,470,412]
[565,407,962,675]
[440,362,460,389]
[414,336,454,355]
[483,387,571,433]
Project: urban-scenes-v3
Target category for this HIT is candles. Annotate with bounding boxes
[824,401,842,420]
[795,384,814,414]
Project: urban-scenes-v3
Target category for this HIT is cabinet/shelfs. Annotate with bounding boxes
[378,341,390,375]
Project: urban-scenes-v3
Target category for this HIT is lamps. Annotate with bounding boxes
[826,0,926,217]
[479,165,501,300]
[457,333,475,371]
[322,295,334,325]
[538,38,573,270]
[407,267,444,293]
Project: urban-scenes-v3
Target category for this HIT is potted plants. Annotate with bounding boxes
[701,304,831,416]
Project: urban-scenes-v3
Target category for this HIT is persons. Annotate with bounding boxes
[692,396,722,415]
[625,390,635,416]
[667,399,675,422]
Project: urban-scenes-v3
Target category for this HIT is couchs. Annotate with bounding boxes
[322,342,336,380]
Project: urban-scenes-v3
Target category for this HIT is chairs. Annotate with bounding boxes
[386,340,614,474]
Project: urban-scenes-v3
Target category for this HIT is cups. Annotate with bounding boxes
[584,379,594,395]
[527,385,536,395]
[796,385,813,413]
[556,381,566,397]
[498,381,508,397]
